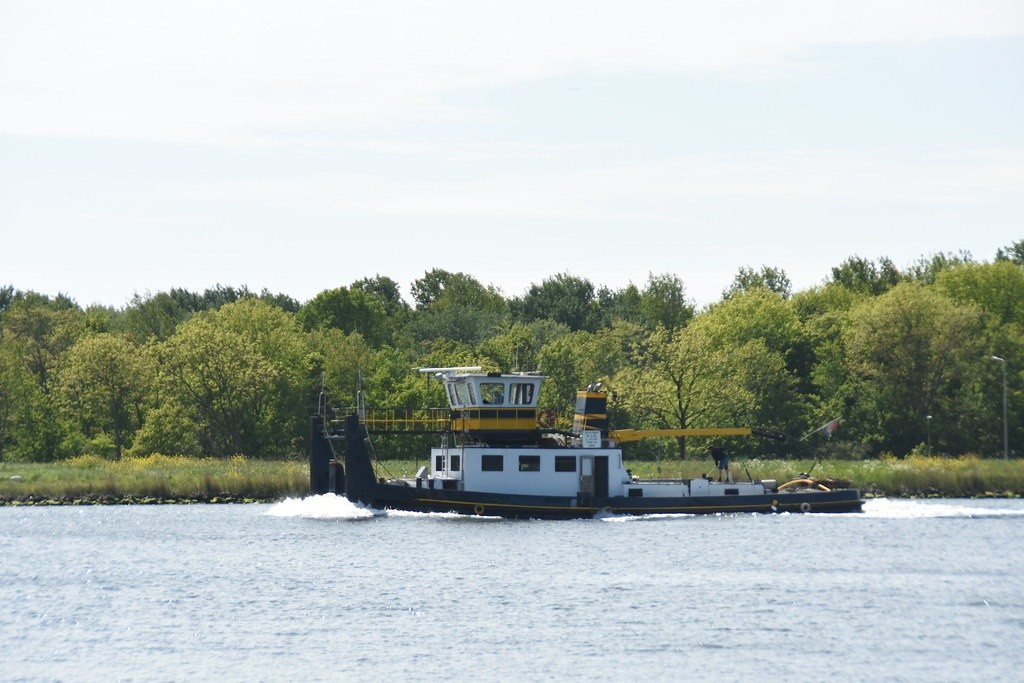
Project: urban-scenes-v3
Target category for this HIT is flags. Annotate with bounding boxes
[820,420,839,441]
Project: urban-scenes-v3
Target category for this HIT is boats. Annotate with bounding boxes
[308,346,866,523]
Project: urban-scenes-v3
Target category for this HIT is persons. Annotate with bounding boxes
[494,391,503,404]
[708,446,729,482]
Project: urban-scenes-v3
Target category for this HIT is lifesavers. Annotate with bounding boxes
[801,502,811,512]
[474,505,484,515]
[538,411,553,428]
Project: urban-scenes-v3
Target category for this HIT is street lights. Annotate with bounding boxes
[926,413,934,458]
[991,354,1009,461]
